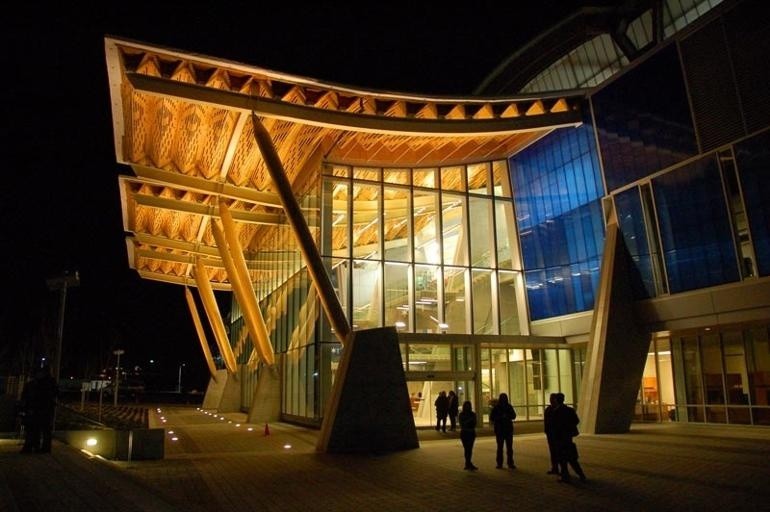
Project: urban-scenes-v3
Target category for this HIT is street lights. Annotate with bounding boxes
[44,269,81,444]
[112,349,125,408]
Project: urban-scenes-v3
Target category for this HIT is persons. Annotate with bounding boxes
[446,391,459,432]
[459,401,479,471]
[543,394,572,477]
[21,368,42,452]
[19,363,59,455]
[489,393,516,469]
[434,391,449,432]
[548,393,588,483]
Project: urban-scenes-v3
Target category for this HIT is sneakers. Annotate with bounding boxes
[436,427,460,433]
[493,465,522,471]
[548,470,590,482]
[463,463,480,474]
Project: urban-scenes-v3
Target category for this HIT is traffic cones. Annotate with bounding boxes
[265,423,270,434]
